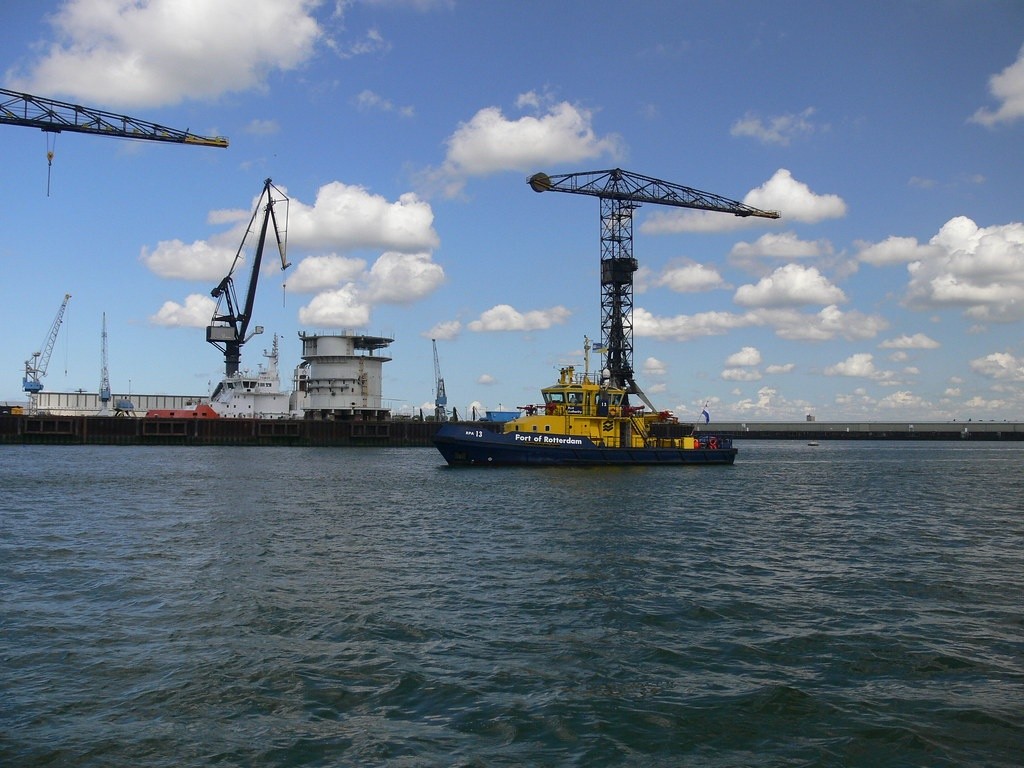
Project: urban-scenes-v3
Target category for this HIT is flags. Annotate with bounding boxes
[702,409,711,425]
[593,342,607,353]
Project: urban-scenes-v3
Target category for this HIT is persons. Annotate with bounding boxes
[571,394,577,403]
[599,438,607,447]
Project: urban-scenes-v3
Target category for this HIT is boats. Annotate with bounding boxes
[434,168,785,463]
